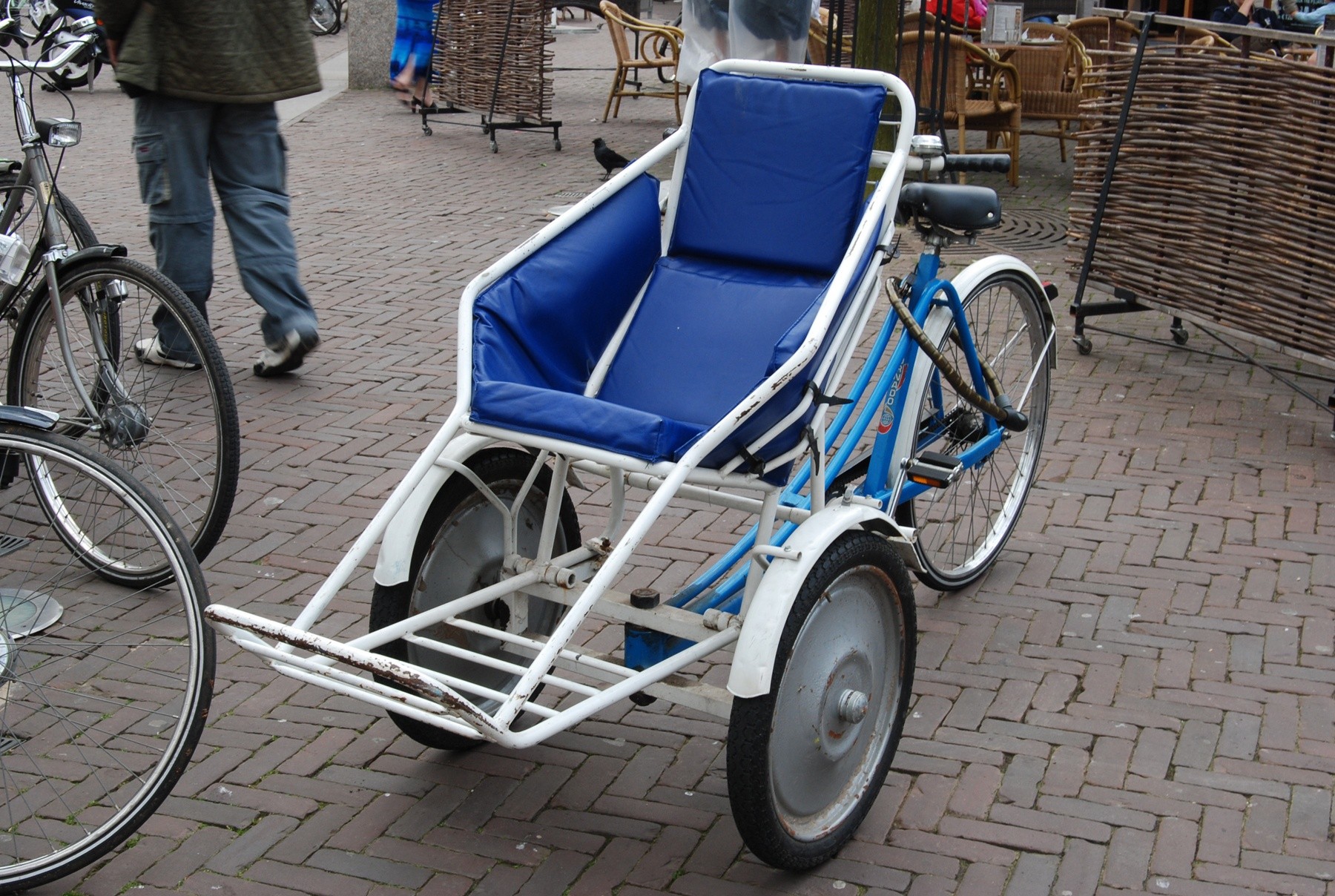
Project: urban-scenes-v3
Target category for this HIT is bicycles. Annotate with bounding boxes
[0,13,241,593]
[0,1,352,444]
[0,403,218,896]
[656,10,684,86]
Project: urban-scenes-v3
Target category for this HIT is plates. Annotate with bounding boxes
[1053,22,1070,26]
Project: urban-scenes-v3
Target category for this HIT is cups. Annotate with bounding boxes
[1070,15,1076,21]
[1057,14,1070,24]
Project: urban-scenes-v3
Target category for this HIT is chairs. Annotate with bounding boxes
[466,72,884,470]
[807,3,1326,191]
[599,0,689,125]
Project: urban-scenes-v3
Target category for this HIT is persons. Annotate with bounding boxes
[910,0,1005,79]
[1205,0,1335,62]
[93,1,325,378]
[384,0,446,113]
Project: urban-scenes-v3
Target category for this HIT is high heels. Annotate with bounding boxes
[389,78,414,105]
[411,97,440,113]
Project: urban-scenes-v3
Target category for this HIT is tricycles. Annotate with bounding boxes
[205,68,1060,875]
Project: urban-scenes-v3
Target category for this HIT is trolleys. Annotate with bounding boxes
[1063,0,1335,446]
[410,1,564,154]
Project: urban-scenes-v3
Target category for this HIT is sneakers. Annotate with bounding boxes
[135,333,203,370]
[253,326,322,376]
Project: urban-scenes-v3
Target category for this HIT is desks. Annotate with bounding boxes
[962,40,1063,142]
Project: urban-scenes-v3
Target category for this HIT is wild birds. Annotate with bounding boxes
[591,137,630,181]
